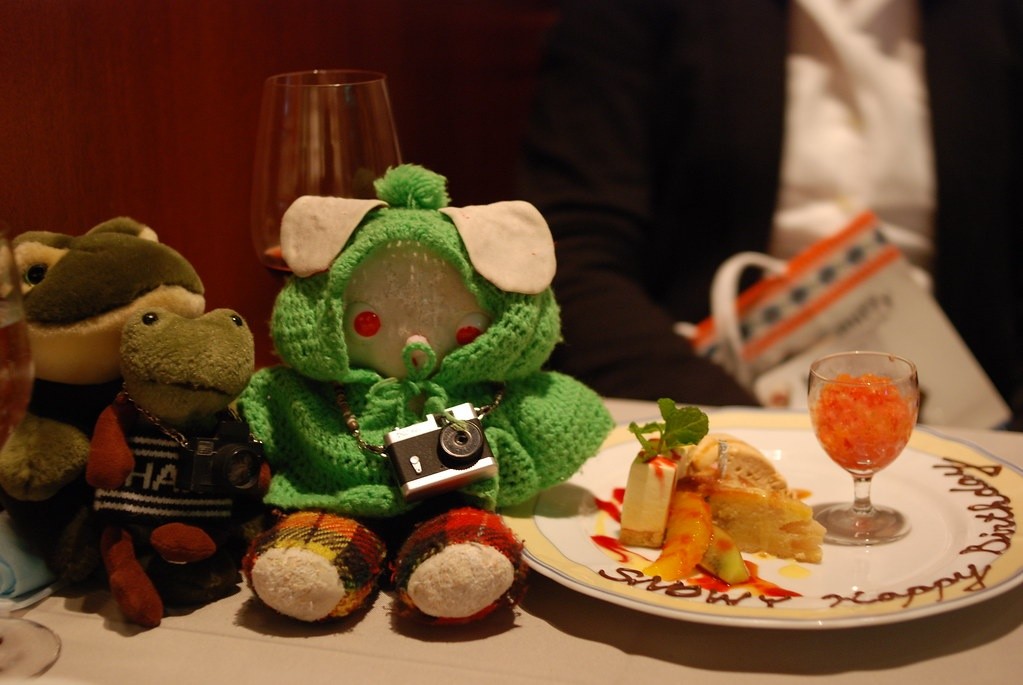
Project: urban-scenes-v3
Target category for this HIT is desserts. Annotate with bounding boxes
[618,398,827,585]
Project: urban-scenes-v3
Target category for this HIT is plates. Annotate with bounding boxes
[496,405,1023,631]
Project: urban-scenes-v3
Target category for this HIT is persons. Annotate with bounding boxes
[519,0,1023,432]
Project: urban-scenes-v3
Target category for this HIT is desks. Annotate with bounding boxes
[10,396,1022,685]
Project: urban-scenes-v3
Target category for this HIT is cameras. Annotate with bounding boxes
[185,433,267,491]
[382,402,498,503]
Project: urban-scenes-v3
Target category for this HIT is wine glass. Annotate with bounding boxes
[808,351,920,545]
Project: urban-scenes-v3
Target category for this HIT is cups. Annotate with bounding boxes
[252,69,405,284]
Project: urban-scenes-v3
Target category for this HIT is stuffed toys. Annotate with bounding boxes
[238,164,618,625]
[88,306,270,630]
[0,217,204,600]
